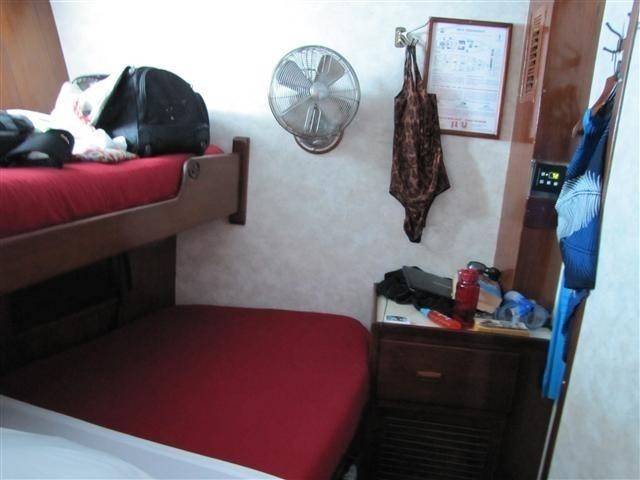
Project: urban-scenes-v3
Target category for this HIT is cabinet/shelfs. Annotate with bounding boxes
[368,282,553,480]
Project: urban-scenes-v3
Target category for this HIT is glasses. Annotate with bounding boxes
[467,261,500,280]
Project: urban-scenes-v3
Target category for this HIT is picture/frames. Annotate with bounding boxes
[423,16,514,140]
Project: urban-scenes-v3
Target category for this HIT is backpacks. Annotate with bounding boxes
[0,109,74,169]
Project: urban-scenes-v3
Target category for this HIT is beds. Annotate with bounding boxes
[0,305,376,480]
[1,135,250,296]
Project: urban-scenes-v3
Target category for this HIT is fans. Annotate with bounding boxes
[268,45,361,155]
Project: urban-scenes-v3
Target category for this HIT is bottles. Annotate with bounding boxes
[419,308,461,330]
[452,269,480,329]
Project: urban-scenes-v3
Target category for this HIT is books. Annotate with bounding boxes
[474,318,530,338]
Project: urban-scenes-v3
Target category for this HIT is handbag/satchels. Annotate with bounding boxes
[71,67,209,158]
